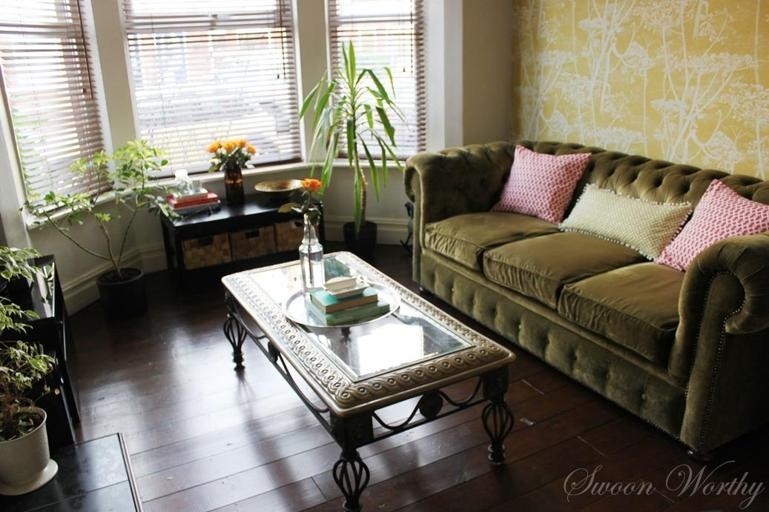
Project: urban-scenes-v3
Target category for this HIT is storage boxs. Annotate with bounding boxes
[178,219,317,271]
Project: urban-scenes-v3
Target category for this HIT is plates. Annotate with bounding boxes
[286,279,402,329]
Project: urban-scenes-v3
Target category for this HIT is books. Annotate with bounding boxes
[304,295,391,325]
[167,187,221,215]
[310,285,379,312]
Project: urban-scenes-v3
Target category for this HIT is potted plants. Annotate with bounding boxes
[298,40,406,252]
[19,139,169,321]
[0,337,63,497]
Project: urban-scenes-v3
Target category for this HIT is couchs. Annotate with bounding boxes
[404,139,769,460]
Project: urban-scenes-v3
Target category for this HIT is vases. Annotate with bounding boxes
[298,212,327,305]
[223,170,245,207]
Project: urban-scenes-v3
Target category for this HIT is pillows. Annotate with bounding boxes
[657,176,769,273]
[491,145,590,225]
[560,183,693,259]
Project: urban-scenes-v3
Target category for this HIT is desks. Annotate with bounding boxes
[157,191,324,285]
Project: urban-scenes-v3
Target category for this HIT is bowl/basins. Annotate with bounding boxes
[254,178,304,200]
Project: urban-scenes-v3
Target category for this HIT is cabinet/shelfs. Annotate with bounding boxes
[0,253,82,447]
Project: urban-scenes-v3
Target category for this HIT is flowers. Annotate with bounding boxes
[279,177,325,301]
[207,137,255,176]
